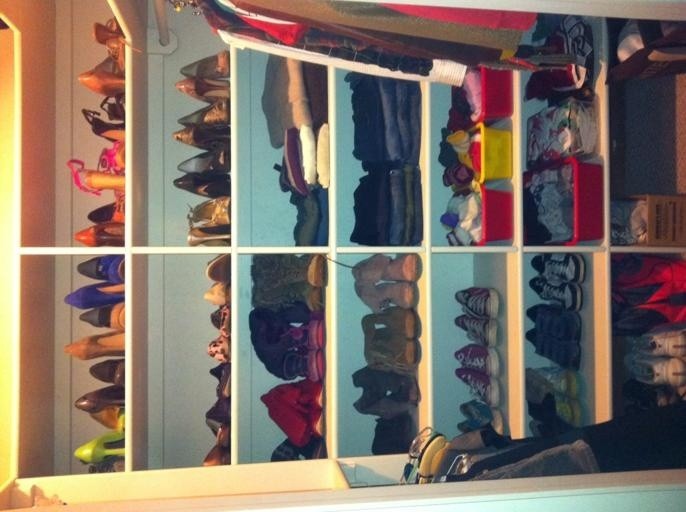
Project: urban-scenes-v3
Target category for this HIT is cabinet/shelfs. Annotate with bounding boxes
[0,1,686,490]
